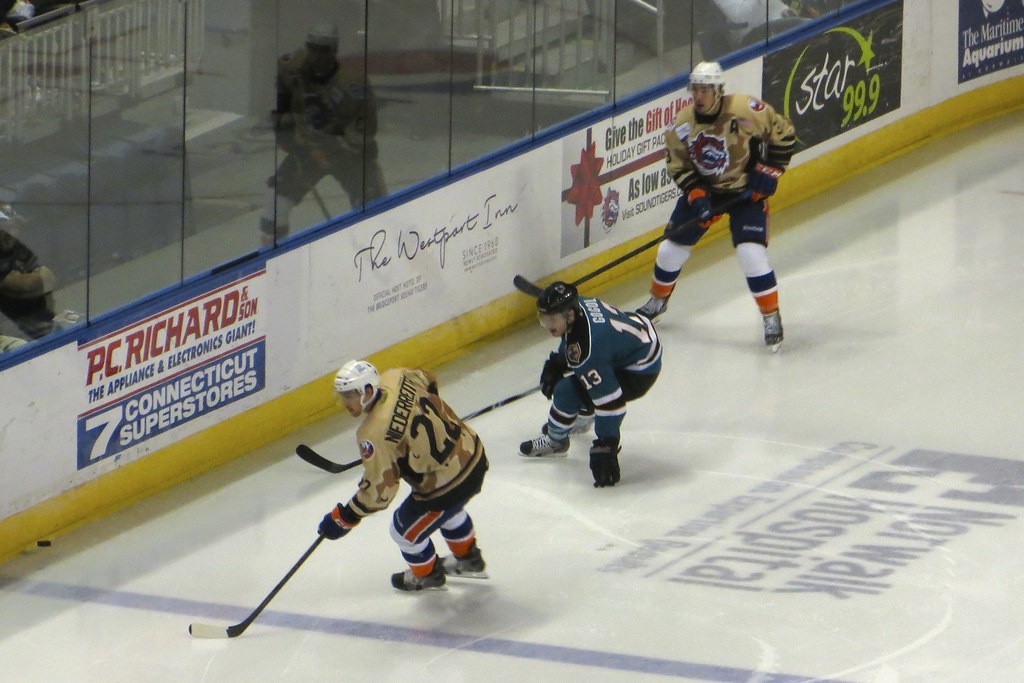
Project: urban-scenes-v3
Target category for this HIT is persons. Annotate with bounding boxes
[521,278,664,492]
[257,21,390,236]
[0,228,59,352]
[318,359,488,591]
[639,61,796,354]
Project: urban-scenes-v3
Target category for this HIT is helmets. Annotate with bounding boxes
[688,62,726,85]
[306,23,339,49]
[333,360,380,405]
[536,281,578,315]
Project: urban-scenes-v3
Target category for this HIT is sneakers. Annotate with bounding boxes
[518,429,570,457]
[439,538,488,579]
[636,282,676,324]
[391,554,448,591]
[762,310,784,353]
[541,416,595,435]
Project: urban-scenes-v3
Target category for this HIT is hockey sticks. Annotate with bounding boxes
[296,385,542,474]
[513,188,752,299]
[188,532,325,639]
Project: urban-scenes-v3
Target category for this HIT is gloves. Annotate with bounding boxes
[273,121,298,152]
[540,351,568,400]
[747,159,785,202]
[589,436,620,488]
[684,180,727,228]
[317,503,362,540]
